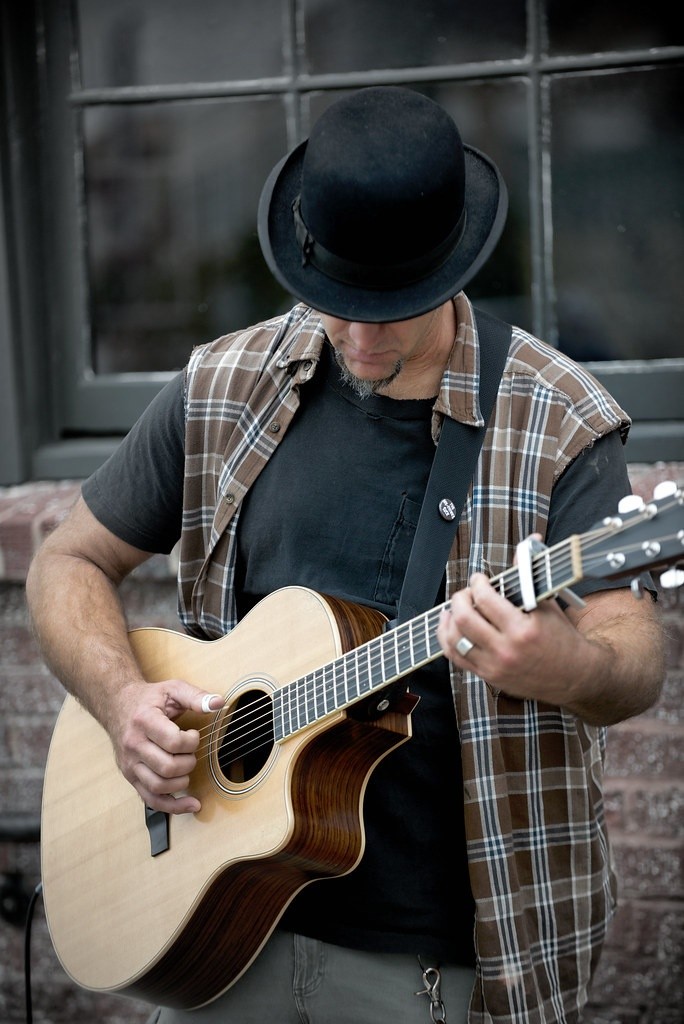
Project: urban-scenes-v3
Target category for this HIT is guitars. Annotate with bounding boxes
[39,476,683,1011]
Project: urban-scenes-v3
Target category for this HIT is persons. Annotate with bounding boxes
[20,84,668,1024]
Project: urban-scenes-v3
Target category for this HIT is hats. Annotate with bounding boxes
[255,85,506,320]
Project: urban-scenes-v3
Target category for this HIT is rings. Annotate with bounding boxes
[456,638,476,657]
